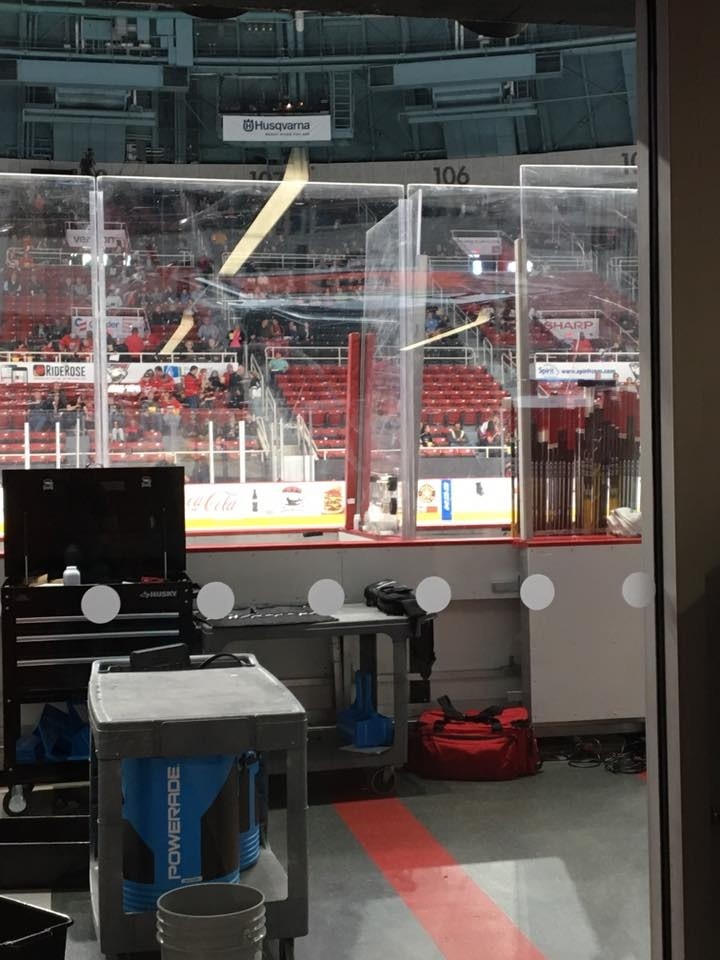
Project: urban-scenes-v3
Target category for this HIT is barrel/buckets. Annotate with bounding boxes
[102,647,273,960]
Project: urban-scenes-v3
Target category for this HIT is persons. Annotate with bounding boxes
[425,313,438,344]
[421,417,501,452]
[27,363,244,442]
[556,332,625,362]
[255,314,313,385]
[3,194,223,361]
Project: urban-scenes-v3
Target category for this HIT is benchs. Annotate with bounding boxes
[0,269,639,461]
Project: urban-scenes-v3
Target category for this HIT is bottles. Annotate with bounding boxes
[62,565,81,586]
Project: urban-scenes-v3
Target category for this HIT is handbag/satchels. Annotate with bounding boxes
[419,695,542,781]
[363,579,436,675]
